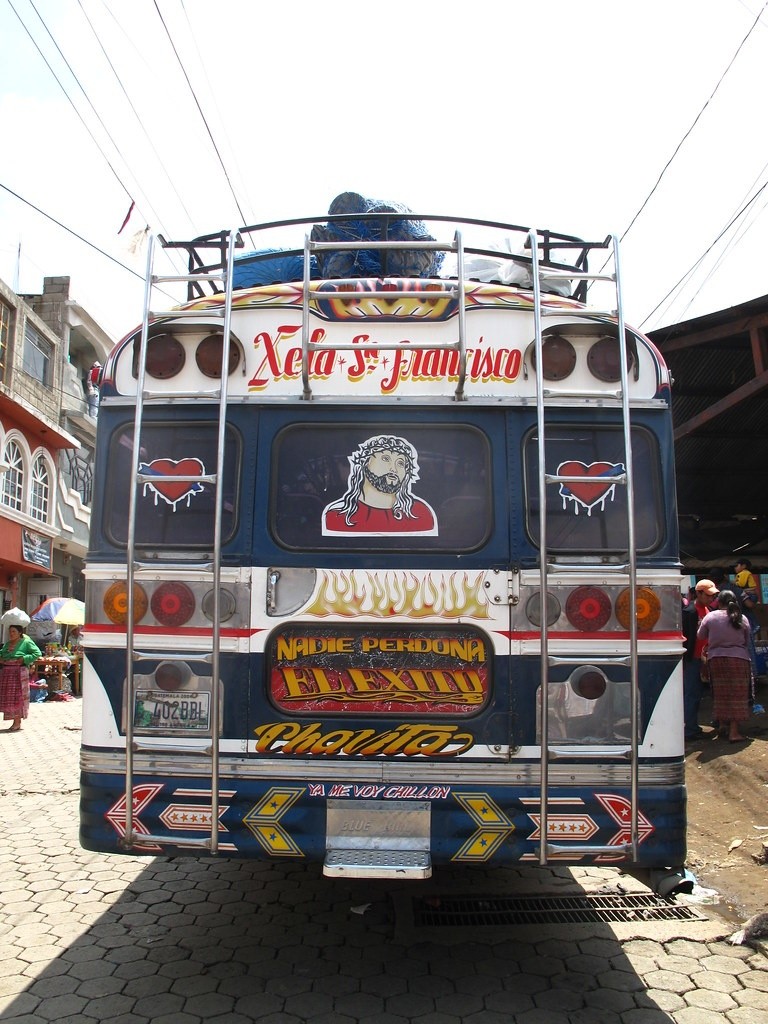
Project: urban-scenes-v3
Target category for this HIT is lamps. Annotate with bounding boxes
[38,430,47,466]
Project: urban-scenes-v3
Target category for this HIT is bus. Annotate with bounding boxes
[77,212,695,901]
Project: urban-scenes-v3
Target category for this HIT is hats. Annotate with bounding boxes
[729,558,751,568]
[695,579,720,595]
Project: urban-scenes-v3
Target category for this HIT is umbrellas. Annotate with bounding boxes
[29,598,85,645]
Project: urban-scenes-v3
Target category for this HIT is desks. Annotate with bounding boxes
[33,657,79,698]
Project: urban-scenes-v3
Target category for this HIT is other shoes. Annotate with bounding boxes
[729,734,747,743]
[5,725,20,732]
[688,732,705,738]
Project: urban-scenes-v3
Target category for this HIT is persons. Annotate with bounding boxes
[29,665,49,689]
[681,559,762,743]
[68,626,84,647]
[0,625,42,732]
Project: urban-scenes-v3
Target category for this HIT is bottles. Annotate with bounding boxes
[45,640,71,657]
